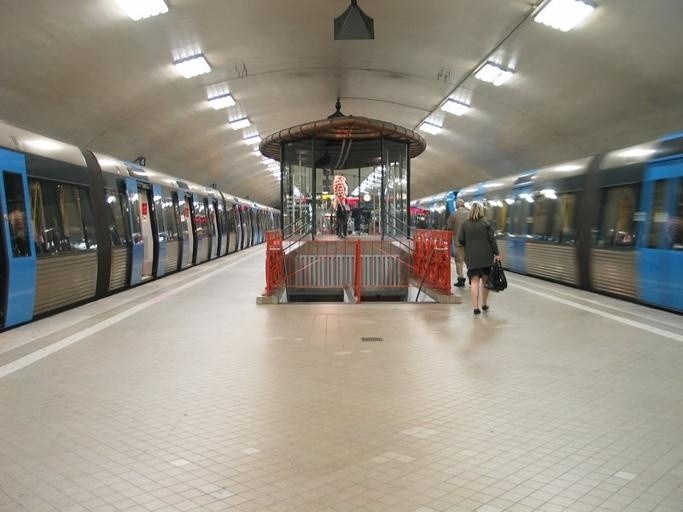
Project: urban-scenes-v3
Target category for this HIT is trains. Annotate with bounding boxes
[0,120,307,329]
[393,132,681,315]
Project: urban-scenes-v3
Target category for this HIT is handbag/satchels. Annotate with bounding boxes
[482,257,507,292]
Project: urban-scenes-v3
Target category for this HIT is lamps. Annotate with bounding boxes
[118,1,305,200]
[418,123,441,136]
[440,98,471,117]
[367,162,408,187]
[531,0,595,34]
[474,61,513,86]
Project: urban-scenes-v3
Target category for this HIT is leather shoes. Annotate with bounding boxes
[474,308,481,314]
[482,305,490,311]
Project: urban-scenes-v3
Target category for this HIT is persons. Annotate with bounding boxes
[456,202,501,314]
[446,198,471,287]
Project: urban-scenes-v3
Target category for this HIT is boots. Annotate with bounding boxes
[453,277,466,286]
